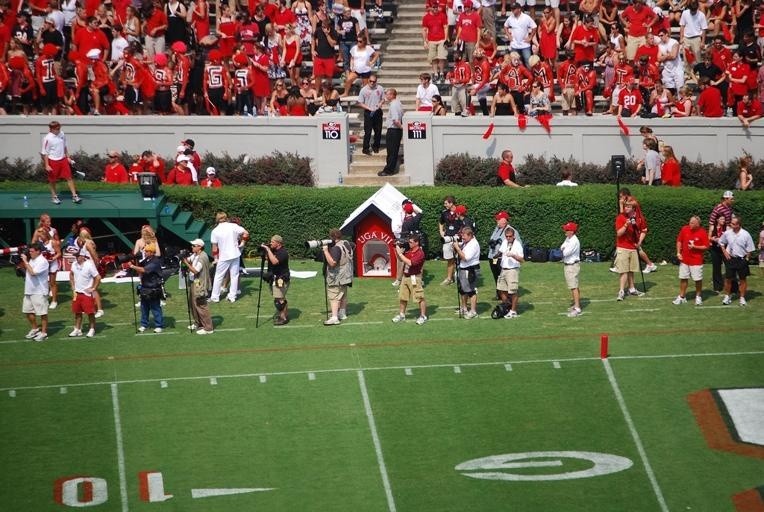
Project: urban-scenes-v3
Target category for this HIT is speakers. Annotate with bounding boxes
[612,155,624,175]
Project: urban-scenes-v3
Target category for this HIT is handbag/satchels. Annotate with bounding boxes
[524,244,563,262]
[580,249,596,261]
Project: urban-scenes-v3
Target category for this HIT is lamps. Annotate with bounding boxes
[136,171,162,198]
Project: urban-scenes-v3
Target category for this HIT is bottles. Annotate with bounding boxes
[67,235,74,246]
[337,172,342,184]
[23,192,29,208]
[252,106,256,116]
[369,106,378,117]
[164,204,168,213]
[243,104,248,116]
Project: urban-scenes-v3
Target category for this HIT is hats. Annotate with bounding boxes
[723,191,735,198]
[9,41,187,70]
[454,205,467,214]
[190,238,204,247]
[106,150,121,158]
[402,198,413,214]
[176,139,215,175]
[72,247,86,255]
[209,45,248,64]
[561,222,577,231]
[495,212,510,220]
[270,234,284,244]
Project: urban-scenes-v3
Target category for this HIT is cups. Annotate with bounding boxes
[263,110,268,116]
[688,240,694,245]
[151,195,155,208]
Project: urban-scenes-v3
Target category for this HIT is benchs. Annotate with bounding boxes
[206,1,396,119]
[435,0,731,117]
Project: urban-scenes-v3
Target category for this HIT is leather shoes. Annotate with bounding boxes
[363,143,379,154]
[378,171,388,176]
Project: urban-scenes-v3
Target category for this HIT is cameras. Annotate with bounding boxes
[488,239,498,249]
[115,252,141,264]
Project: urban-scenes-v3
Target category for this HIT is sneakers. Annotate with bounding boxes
[440,279,453,286]
[226,294,234,302]
[628,288,645,297]
[71,195,82,203]
[568,307,581,317]
[273,315,292,325]
[210,298,219,302]
[642,263,657,273]
[323,313,347,325]
[673,290,746,307]
[392,313,406,322]
[25,289,105,343]
[456,309,518,319]
[135,299,211,334]
[392,279,401,286]
[416,313,428,324]
[51,195,60,204]
[609,266,620,273]
[617,292,624,301]
